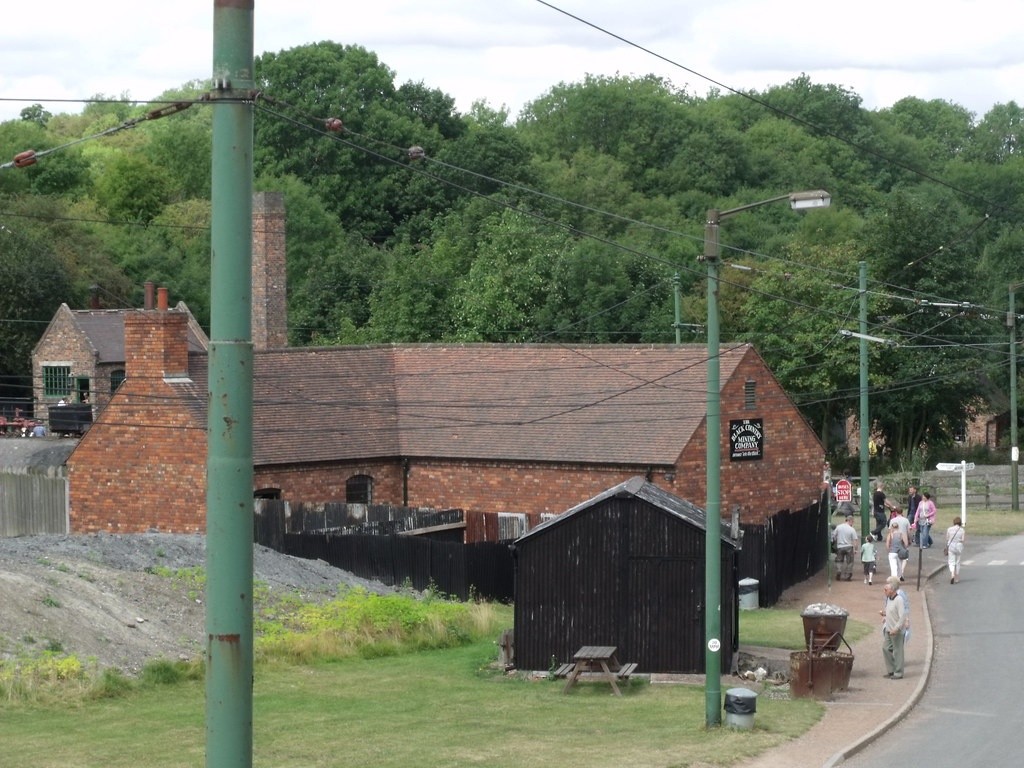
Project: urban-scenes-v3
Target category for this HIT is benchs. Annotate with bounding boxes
[554,661,575,676]
[617,661,638,680]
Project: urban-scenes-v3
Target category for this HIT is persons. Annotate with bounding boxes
[879,577,912,680]
[946,517,965,584]
[32,420,46,438]
[888,507,913,581]
[57,396,69,407]
[886,523,907,577]
[831,516,858,581]
[907,486,934,548]
[914,492,936,549]
[860,535,878,585]
[869,436,884,460]
[870,483,897,541]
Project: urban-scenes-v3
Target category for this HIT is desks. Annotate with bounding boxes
[565,646,624,696]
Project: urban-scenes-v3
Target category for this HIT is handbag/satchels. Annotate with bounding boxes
[898,533,909,559]
[943,544,948,555]
[926,516,936,526]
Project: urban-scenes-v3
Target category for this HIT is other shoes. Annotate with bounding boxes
[891,676,903,679]
[870,532,878,541]
[928,543,932,547]
[900,576,904,581]
[913,544,920,547]
[950,576,954,583]
[923,546,927,548]
[883,674,893,678]
[864,579,867,584]
[868,580,872,585]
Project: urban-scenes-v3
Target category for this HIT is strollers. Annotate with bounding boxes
[887,506,916,541]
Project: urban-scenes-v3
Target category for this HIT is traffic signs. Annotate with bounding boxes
[937,463,976,472]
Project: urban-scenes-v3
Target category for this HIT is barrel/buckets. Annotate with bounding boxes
[789,649,834,700]
[818,632,855,693]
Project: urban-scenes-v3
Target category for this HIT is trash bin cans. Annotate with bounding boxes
[725,687,759,730]
[34,427,45,438]
[739,576,760,609]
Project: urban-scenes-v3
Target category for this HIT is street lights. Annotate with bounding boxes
[705,190,831,728]
